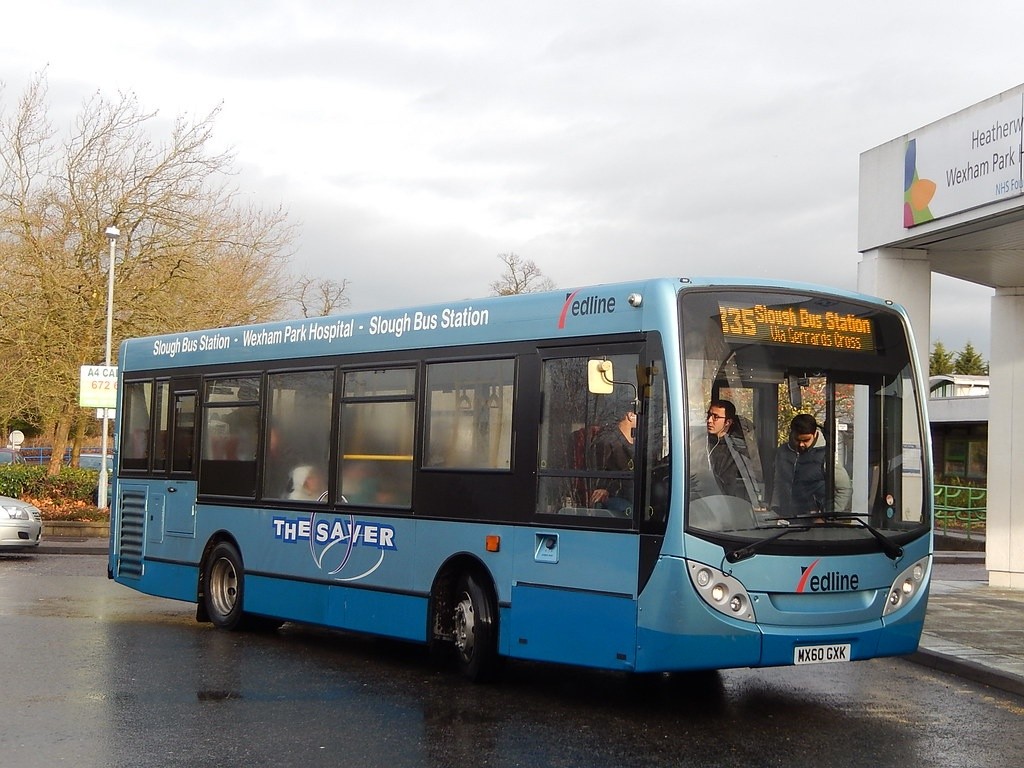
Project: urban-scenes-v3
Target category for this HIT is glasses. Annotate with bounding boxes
[706,412,726,421]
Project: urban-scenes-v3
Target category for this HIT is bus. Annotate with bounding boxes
[106,276,934,681]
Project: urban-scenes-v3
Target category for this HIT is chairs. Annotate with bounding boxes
[203,419,231,460]
[131,428,166,458]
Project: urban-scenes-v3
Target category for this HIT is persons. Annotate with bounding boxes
[576,397,666,521]
[657,400,763,525]
[770,414,852,523]
[221,386,454,506]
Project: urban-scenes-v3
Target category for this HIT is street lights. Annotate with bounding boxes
[97,225,120,511]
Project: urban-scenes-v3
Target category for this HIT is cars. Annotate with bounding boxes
[1,493,45,549]
